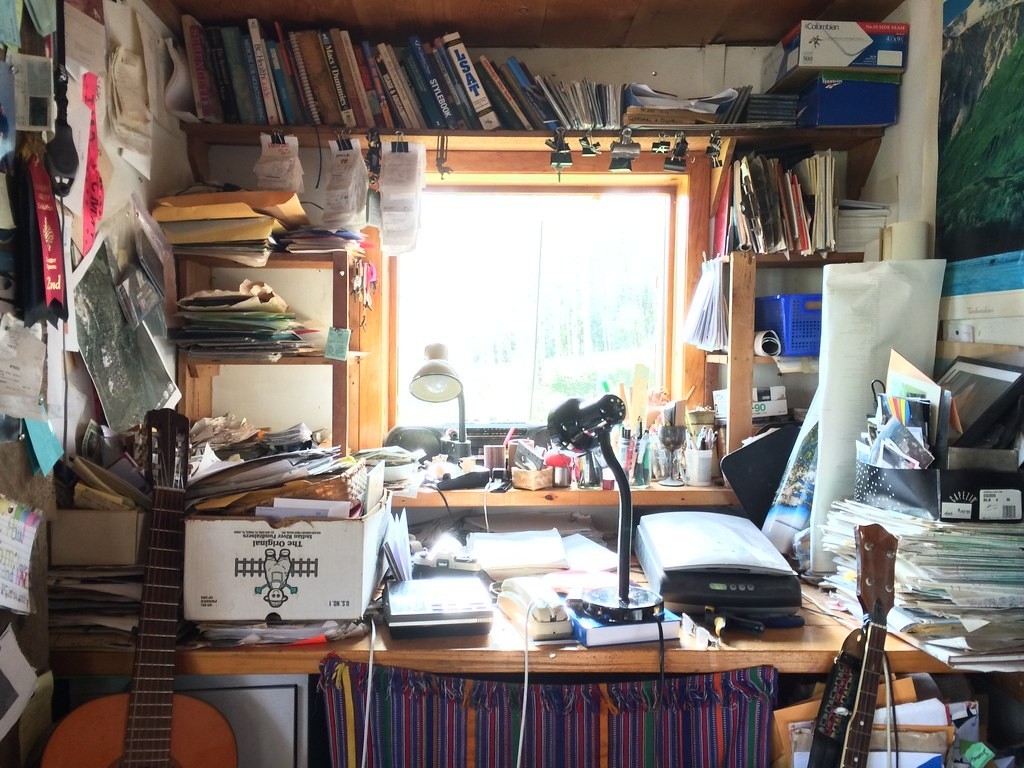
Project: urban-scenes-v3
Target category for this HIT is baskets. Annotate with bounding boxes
[755,293,822,357]
[227,456,368,517]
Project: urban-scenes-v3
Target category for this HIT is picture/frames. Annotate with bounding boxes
[936,355,1024,448]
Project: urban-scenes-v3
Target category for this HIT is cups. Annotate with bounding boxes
[619,437,652,489]
[554,465,572,488]
[688,410,720,479]
[676,448,713,487]
[508,438,534,467]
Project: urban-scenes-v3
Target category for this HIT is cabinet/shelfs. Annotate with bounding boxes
[52,0,1024,768]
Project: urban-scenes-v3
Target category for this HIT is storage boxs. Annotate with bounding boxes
[712,386,788,418]
[799,72,899,129]
[511,466,552,490]
[788,723,958,767]
[761,21,909,94]
[49,507,147,566]
[185,490,391,619]
[854,459,1024,523]
[756,296,822,357]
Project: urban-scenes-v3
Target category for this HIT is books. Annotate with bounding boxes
[861,377,953,468]
[176,15,801,129]
[731,147,887,257]
[549,578,683,649]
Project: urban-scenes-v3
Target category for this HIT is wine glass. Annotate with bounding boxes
[658,425,686,487]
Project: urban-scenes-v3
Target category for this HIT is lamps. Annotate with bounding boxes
[547,395,665,624]
[411,344,470,464]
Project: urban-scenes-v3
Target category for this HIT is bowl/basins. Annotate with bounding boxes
[365,461,419,484]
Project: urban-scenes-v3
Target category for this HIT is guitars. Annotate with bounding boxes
[840,521,902,767]
[27,408,242,768]
